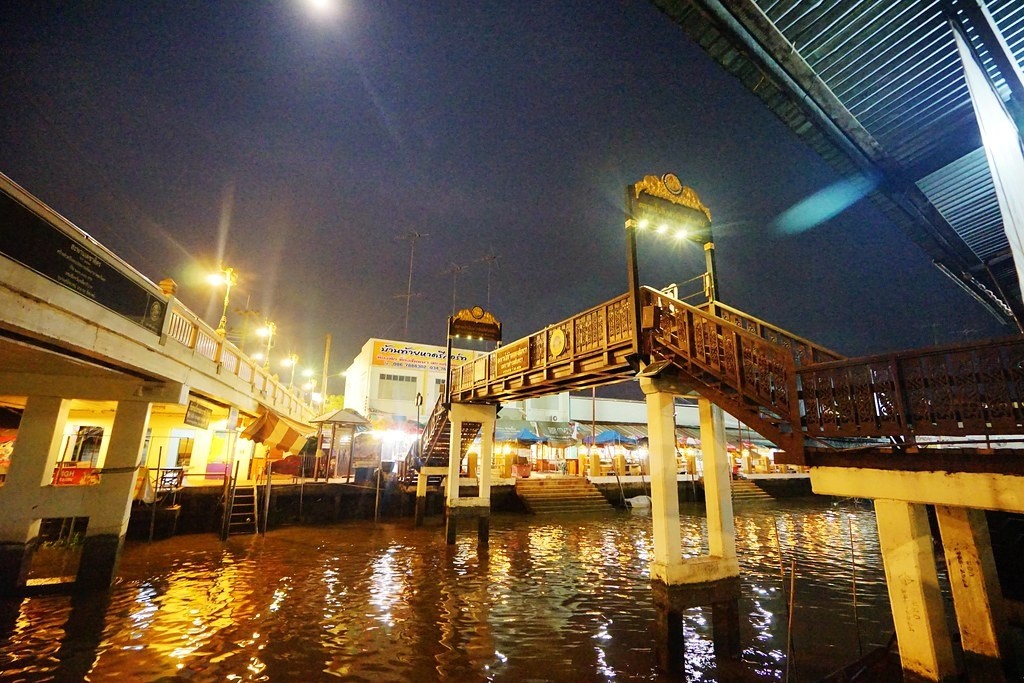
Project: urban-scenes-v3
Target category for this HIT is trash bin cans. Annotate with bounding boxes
[352,467,378,483]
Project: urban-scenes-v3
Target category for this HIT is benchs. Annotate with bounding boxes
[256,473,300,484]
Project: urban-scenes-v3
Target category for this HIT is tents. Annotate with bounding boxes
[493,427,547,472]
[583,430,635,458]
[633,435,737,459]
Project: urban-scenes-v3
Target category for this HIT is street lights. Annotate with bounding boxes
[303,368,316,405]
[319,371,346,417]
[255,321,275,369]
[283,352,300,392]
[209,266,237,338]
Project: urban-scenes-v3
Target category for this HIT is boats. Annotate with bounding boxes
[623,495,652,509]
